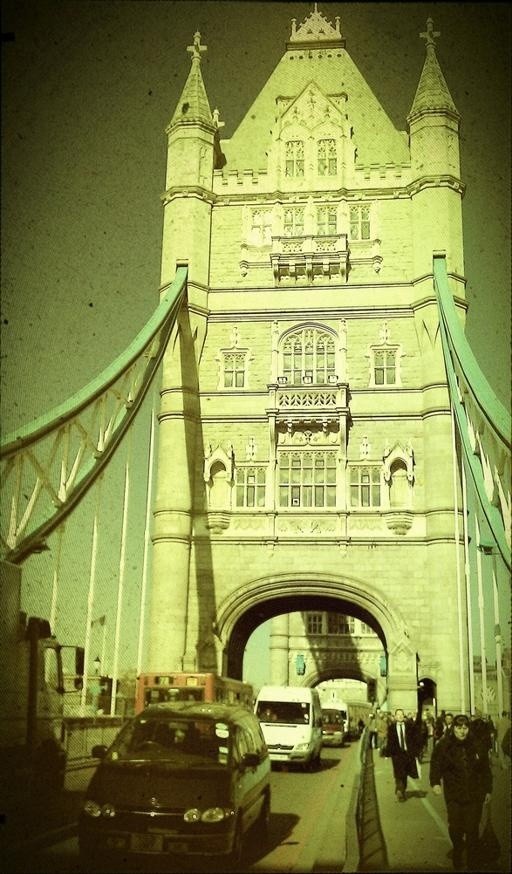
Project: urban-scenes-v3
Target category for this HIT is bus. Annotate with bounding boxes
[133,670,257,739]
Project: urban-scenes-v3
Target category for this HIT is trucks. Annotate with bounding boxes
[246,688,321,771]
[0,561,89,874]
[322,699,349,741]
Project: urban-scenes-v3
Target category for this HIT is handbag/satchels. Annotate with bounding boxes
[476,804,501,862]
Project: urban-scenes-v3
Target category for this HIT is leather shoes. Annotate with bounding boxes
[396,790,405,802]
[453,840,465,870]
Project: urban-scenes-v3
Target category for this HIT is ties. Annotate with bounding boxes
[400,724,404,752]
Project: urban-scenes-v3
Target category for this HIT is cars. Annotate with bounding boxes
[77,701,275,871]
[321,709,345,747]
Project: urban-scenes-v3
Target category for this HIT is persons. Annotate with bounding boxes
[259,707,279,722]
[384,707,416,801]
[428,713,492,872]
[364,706,512,772]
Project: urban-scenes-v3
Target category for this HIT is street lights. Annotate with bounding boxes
[93,656,102,716]
